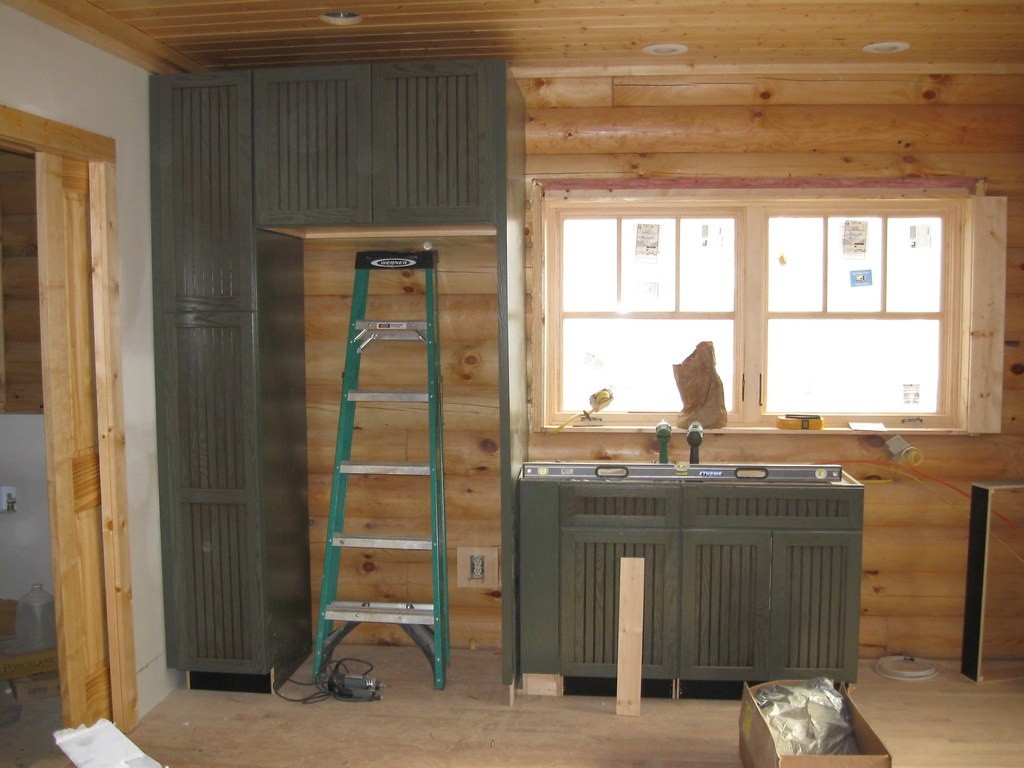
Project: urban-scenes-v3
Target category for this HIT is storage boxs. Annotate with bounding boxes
[10,668,61,704]
[739,678,895,768]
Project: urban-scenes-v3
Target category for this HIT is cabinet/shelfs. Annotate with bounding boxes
[250,58,530,241]
[519,480,864,698]
[145,58,314,698]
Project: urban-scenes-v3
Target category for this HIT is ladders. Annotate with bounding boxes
[313,250,452,689]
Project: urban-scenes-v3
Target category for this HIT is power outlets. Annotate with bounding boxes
[470,556,486,580]
